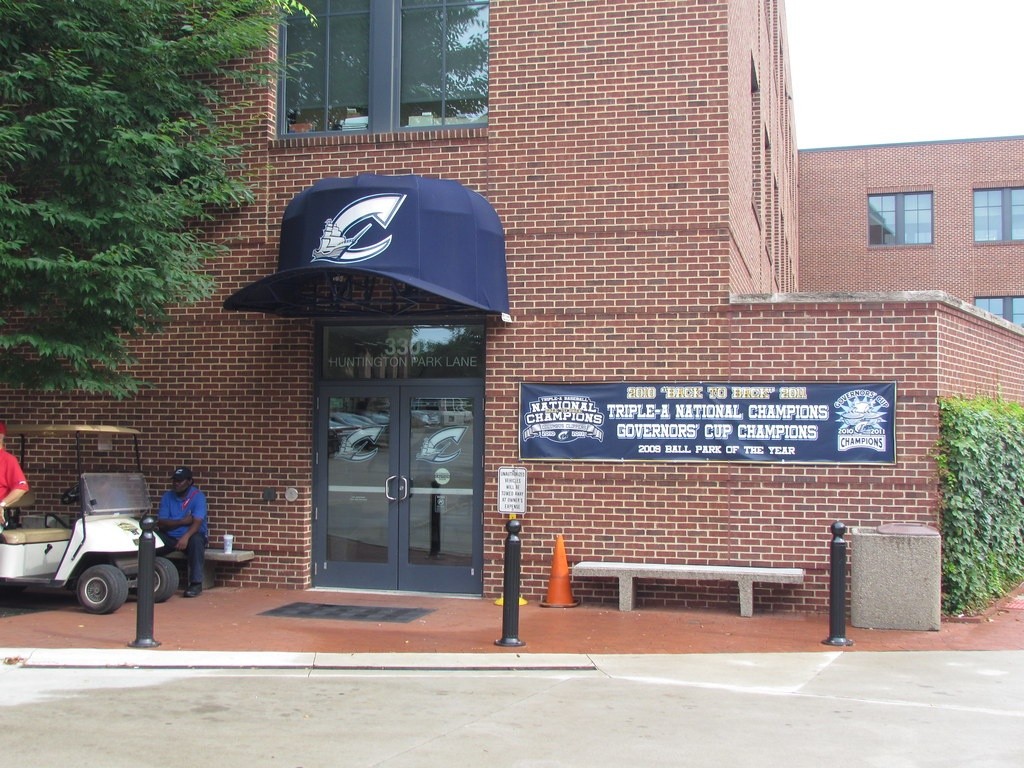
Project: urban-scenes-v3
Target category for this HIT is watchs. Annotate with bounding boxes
[0,501,7,508]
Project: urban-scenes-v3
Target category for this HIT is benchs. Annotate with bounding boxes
[162,549,255,589]
[572,562,804,617]
[0,513,73,544]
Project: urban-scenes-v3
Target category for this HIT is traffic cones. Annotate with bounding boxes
[539,534,580,608]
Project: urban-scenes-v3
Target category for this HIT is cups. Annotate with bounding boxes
[223,534,233,554]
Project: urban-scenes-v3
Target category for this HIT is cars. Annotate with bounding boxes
[328,412,390,443]
[410,410,441,427]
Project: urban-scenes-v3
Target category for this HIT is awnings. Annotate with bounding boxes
[223,173,510,315]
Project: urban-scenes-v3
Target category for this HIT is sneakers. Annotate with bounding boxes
[184,584,202,597]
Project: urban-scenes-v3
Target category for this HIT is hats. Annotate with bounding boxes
[170,468,192,480]
[0,422,6,434]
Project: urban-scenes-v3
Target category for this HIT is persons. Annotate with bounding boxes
[0,424,30,538]
[153,468,208,597]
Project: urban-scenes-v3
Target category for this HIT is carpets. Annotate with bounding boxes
[257,601,438,623]
